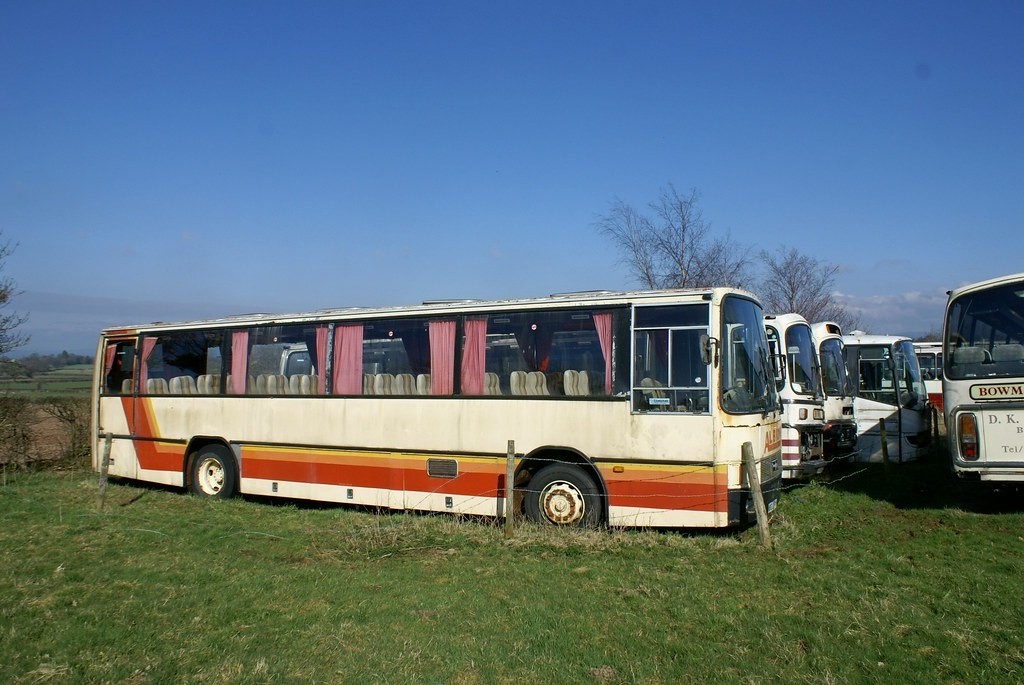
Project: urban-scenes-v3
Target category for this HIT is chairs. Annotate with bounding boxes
[954,347,986,365]
[861,361,896,405]
[121,372,709,412]
[992,344,1024,362]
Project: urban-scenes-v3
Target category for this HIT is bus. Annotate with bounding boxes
[913,341,946,417]
[940,269,1024,496]
[812,319,858,481]
[87,280,779,536]
[843,333,926,466]
[762,310,824,487]
